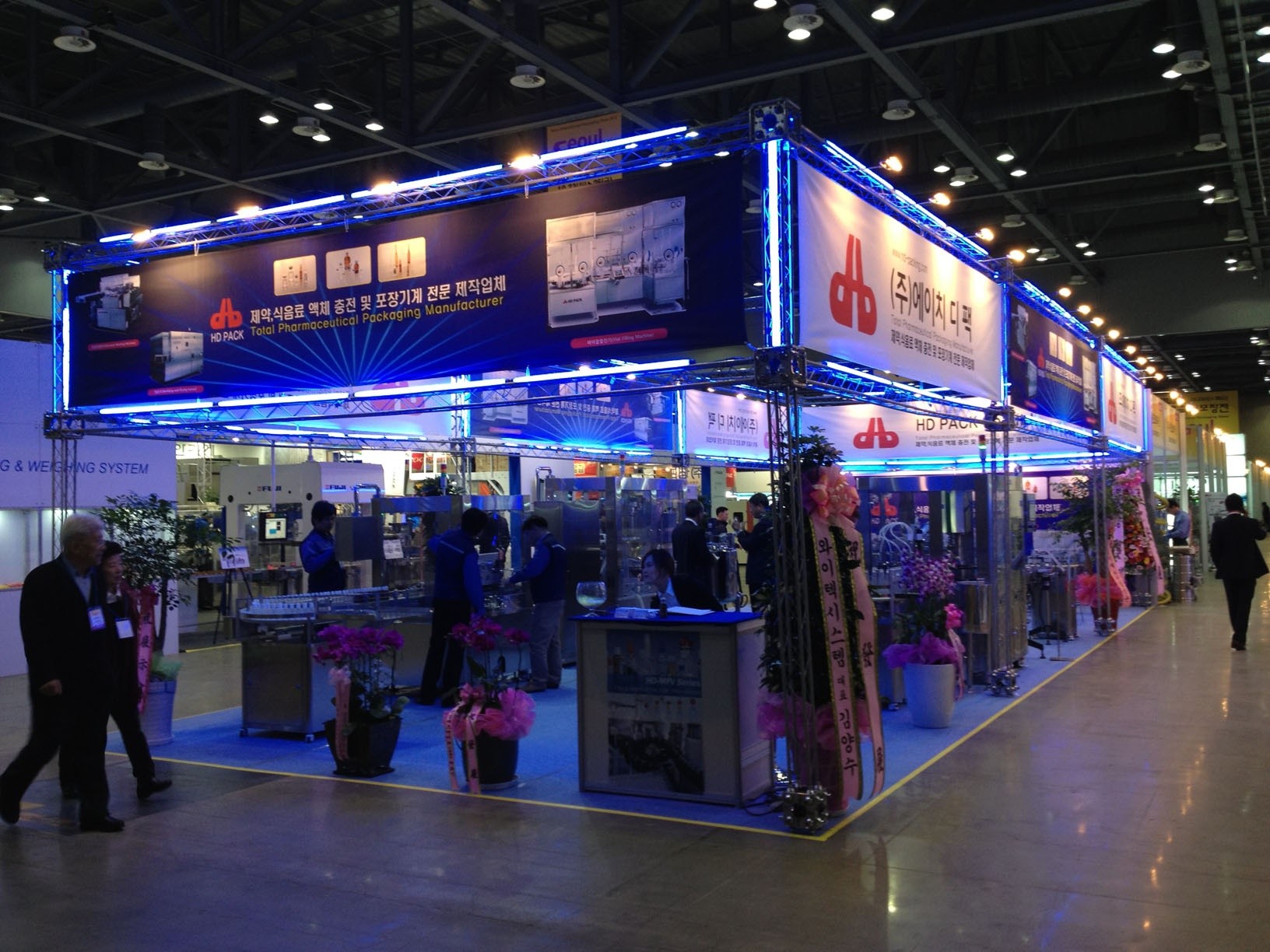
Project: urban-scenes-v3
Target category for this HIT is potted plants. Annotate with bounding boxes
[1054,453,1140,632]
[82,490,244,747]
[748,425,878,802]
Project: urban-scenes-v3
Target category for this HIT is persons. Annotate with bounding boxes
[732,493,781,614]
[299,501,347,592]
[0,512,173,834]
[641,548,725,610]
[505,516,566,694]
[1162,499,1191,546]
[417,508,490,707]
[671,500,716,592]
[1207,494,1270,651]
[716,507,743,594]
[479,511,510,591]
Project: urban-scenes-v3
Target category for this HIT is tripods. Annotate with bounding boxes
[212,570,252,644]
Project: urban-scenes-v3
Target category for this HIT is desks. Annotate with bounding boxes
[572,608,777,809]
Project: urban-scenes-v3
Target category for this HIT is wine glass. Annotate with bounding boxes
[576,582,607,618]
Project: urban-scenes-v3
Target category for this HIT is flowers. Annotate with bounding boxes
[308,622,413,721]
[447,615,530,716]
[1123,513,1155,567]
[896,545,964,660]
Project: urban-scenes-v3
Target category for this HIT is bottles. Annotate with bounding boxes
[659,597,668,618]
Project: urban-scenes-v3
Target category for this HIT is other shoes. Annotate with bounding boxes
[518,682,544,693]
[78,814,125,831]
[416,695,455,709]
[0,778,20,824]
[137,777,173,799]
[1230,640,1247,650]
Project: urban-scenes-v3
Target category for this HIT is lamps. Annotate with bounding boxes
[823,359,989,410]
[92,357,700,416]
[840,155,1201,418]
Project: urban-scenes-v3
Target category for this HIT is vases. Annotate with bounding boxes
[461,729,519,790]
[902,661,956,728]
[324,714,405,776]
[1124,571,1144,606]
